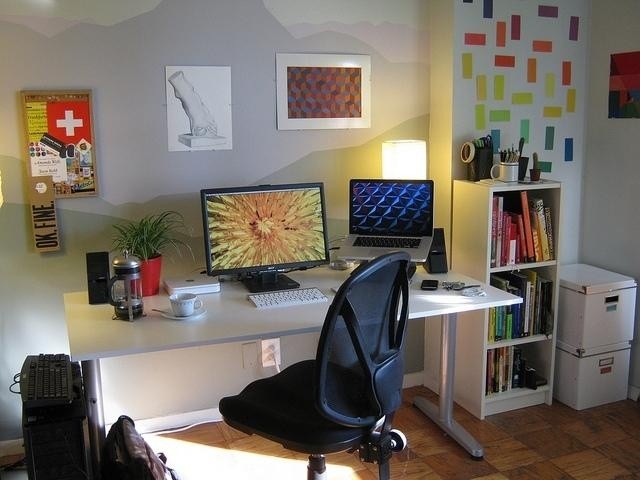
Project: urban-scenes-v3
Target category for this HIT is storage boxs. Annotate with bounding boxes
[556,264,639,358]
[553,347,633,412]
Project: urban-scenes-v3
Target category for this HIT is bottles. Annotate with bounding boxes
[106,249,144,320]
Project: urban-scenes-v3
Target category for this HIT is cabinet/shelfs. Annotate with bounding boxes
[449,176,565,422]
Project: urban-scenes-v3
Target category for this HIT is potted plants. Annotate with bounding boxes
[109,206,198,298]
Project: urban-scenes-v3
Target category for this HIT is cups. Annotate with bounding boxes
[490,162,519,183]
[467,146,494,181]
[529,169,541,181]
[518,157,529,179]
[168,292,203,317]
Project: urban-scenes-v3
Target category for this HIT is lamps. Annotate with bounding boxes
[379,136,430,181]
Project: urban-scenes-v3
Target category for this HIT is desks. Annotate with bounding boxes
[61,263,526,463]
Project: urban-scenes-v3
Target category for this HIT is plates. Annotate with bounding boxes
[159,307,207,321]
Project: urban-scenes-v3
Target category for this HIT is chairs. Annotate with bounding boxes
[216,249,418,479]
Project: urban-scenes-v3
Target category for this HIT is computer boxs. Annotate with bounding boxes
[22,361,90,480]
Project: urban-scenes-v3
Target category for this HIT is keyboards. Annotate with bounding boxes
[247,286,328,309]
[19,353,72,417]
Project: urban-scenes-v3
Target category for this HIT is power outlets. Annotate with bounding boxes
[260,337,283,368]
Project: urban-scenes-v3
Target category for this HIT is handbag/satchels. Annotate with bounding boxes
[102,415,178,479]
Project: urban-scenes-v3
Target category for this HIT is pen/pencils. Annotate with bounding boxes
[500,144,521,163]
[474,137,493,147]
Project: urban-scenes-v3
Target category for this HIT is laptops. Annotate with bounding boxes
[337,178,434,263]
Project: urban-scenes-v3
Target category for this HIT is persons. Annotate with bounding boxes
[169,71,216,138]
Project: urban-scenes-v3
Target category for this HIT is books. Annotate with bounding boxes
[485,190,553,397]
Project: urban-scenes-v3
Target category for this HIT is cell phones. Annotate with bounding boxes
[421,279,439,289]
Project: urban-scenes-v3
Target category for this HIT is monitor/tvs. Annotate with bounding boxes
[200,182,330,292]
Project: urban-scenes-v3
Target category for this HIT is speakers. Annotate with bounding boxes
[85,251,110,305]
[424,228,448,274]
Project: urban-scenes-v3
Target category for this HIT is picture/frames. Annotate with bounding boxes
[20,87,101,201]
[274,51,373,131]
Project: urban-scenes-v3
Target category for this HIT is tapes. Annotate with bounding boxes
[460,142,475,165]
